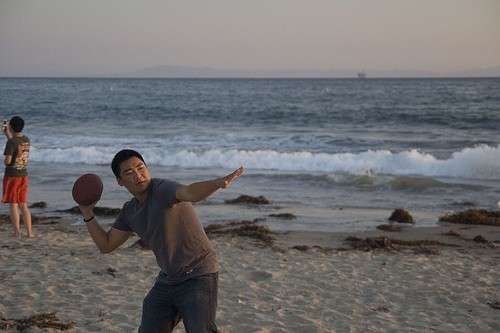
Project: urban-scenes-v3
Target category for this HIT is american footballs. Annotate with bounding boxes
[71,172,104,206]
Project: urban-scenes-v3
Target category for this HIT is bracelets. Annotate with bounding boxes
[84,213,94,222]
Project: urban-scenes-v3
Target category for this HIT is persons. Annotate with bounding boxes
[2,115,35,239]
[78,149,246,333]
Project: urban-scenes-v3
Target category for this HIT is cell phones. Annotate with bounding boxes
[2,119,7,130]
